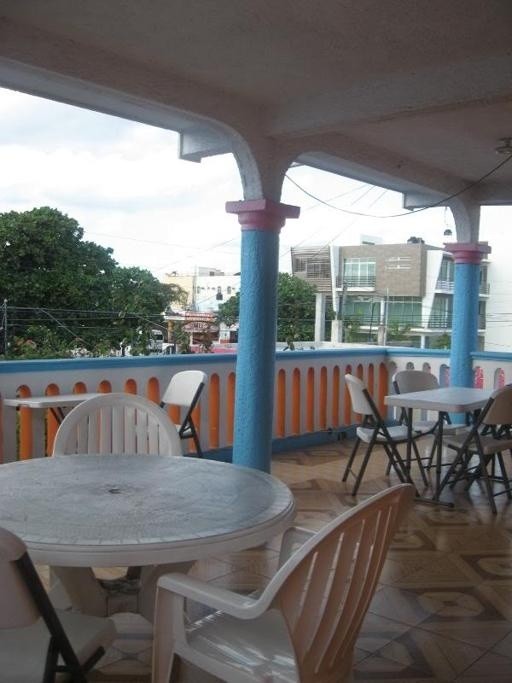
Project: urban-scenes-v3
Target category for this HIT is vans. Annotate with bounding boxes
[138,328,165,352]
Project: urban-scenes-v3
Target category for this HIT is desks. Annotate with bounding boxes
[4,390,112,460]
[1,447,299,681]
[375,379,501,495]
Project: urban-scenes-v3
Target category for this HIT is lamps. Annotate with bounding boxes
[495,135,511,156]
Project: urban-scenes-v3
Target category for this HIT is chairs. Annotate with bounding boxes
[392,368,473,486]
[146,481,413,682]
[1,524,123,680]
[439,377,510,516]
[144,367,209,461]
[48,392,184,608]
[339,370,430,497]
